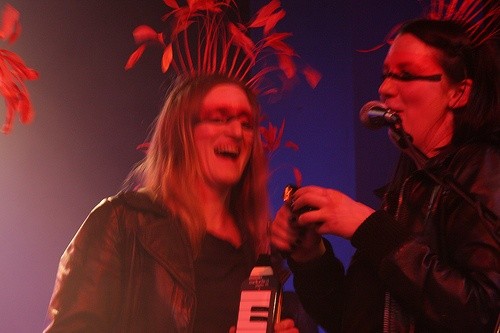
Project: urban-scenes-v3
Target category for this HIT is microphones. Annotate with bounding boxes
[359,101,403,130]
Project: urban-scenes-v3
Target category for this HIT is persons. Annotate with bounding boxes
[269,16,500,333]
[44,70,319,333]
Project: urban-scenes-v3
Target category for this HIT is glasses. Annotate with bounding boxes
[193,111,255,133]
